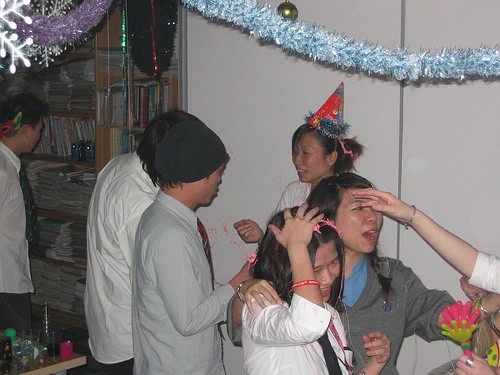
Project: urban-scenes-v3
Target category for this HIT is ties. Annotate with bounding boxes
[20,162,41,247]
[196,217,224,341]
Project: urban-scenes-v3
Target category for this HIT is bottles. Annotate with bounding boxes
[40,302,50,344]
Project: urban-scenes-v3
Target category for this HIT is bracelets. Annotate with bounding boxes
[404,204,416,230]
[488,319,500,339]
[477,297,488,314]
[291,280,319,291]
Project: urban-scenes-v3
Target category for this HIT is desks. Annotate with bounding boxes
[0,329,88,375]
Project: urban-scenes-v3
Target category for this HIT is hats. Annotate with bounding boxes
[154,120,228,183]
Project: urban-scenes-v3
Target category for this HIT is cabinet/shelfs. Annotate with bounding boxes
[17,0,180,332]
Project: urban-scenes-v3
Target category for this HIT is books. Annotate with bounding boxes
[4,40,180,346]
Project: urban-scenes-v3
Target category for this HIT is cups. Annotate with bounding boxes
[0,336,13,374]
[60,341,73,357]
[47,332,62,357]
[14,341,34,372]
[24,328,40,346]
[4,328,17,342]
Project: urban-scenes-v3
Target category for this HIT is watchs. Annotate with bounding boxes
[235,280,248,304]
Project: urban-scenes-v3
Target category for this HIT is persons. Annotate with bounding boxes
[133,109,260,375]
[84,118,161,375]
[226,171,500,375]
[0,94,47,375]
[233,82,367,248]
[241,203,392,375]
[351,188,500,375]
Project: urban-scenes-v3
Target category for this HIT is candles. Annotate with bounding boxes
[58,340,74,358]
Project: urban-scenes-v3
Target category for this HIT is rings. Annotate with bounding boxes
[465,356,474,367]
